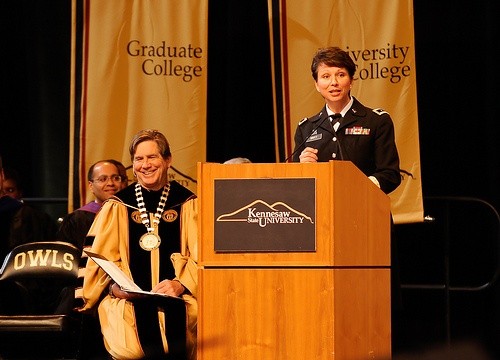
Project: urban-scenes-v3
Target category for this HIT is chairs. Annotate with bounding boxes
[0,241,81,360]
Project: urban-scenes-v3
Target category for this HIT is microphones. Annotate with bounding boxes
[284,114,337,163]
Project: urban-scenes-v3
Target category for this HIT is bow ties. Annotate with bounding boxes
[330,112,344,126]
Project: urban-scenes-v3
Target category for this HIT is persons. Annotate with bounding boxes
[0,159,127,360]
[293,47,401,194]
[76,129,198,360]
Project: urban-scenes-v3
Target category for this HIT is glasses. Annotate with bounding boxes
[89,176,121,184]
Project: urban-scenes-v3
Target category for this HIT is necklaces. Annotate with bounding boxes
[135,181,170,251]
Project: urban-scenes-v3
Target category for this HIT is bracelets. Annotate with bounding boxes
[109,283,116,298]
